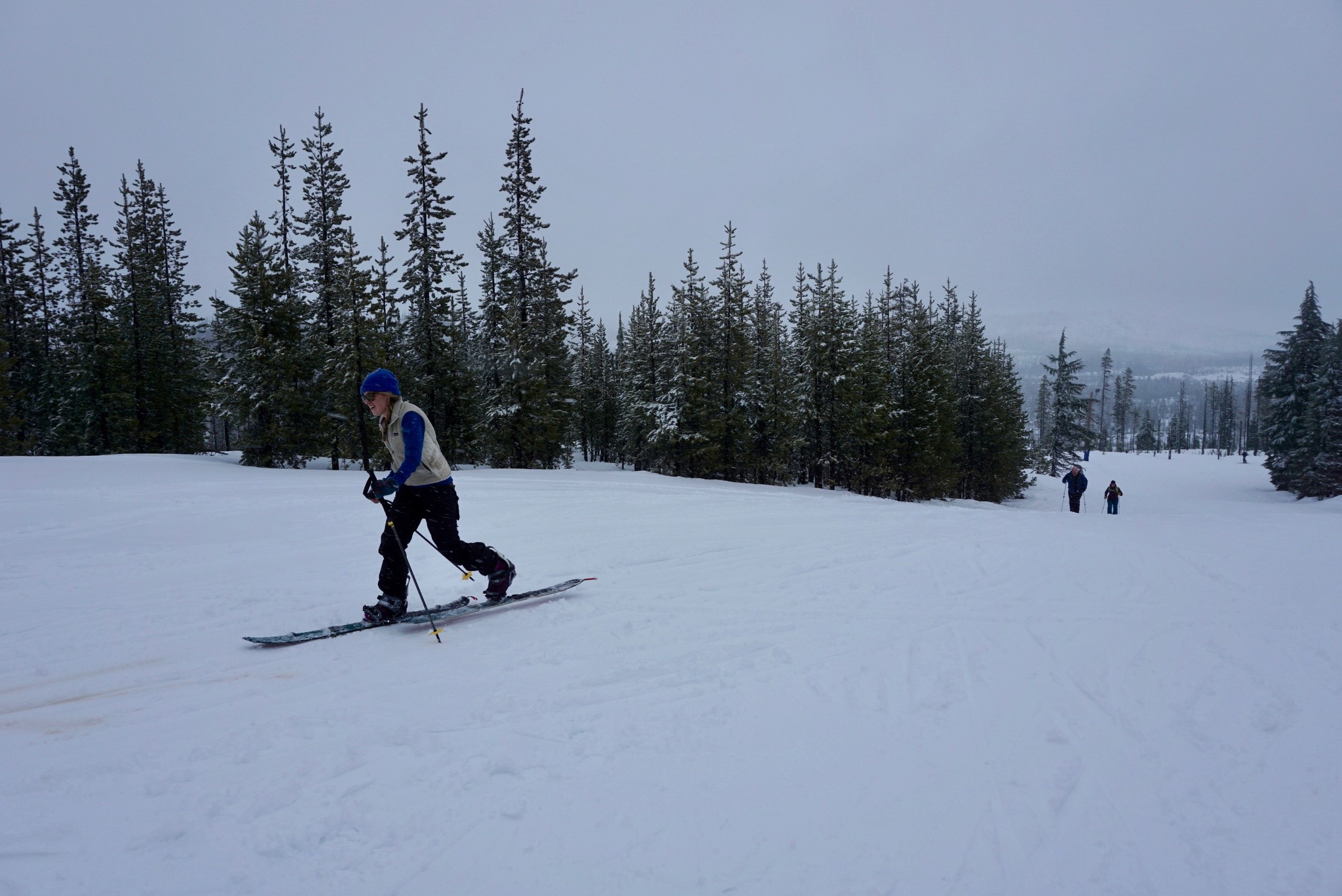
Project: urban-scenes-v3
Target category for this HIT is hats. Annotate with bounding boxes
[360,369,401,398]
[1111,480,1115,484]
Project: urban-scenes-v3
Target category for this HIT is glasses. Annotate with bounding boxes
[361,391,378,402]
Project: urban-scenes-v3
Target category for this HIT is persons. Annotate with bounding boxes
[1242,450,1248,464]
[1062,467,1088,513]
[1104,480,1123,514]
[360,368,517,623]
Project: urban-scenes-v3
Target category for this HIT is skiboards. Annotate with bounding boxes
[240,578,584,644]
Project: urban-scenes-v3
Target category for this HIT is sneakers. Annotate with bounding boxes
[483,561,516,599]
[365,600,406,621]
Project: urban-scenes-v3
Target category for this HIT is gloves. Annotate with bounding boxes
[1104,496,1107,499]
[1118,492,1121,495]
